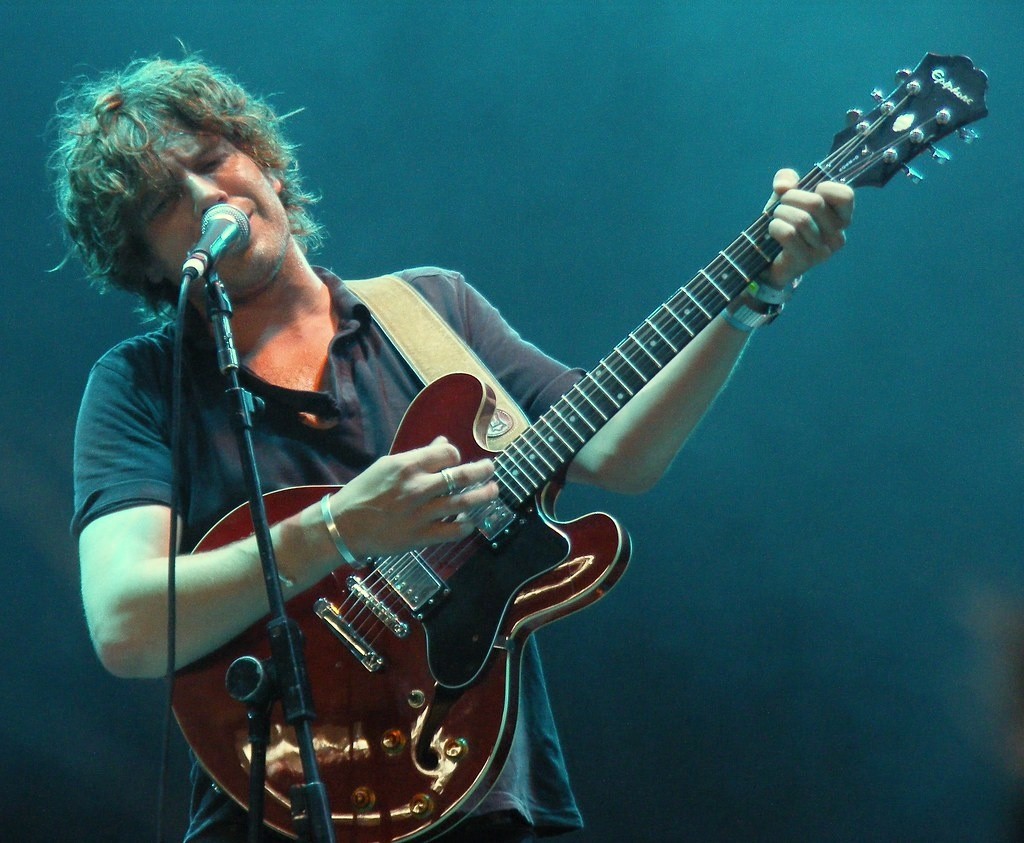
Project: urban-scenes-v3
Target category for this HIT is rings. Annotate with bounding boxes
[442,468,455,490]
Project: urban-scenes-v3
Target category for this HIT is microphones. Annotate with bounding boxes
[182,204,251,280]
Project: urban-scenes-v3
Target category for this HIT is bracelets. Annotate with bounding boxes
[728,302,784,329]
[321,492,366,569]
[748,277,803,304]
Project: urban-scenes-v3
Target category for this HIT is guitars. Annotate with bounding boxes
[164,48,994,843]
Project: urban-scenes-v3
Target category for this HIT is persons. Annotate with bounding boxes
[57,57,854,843]
